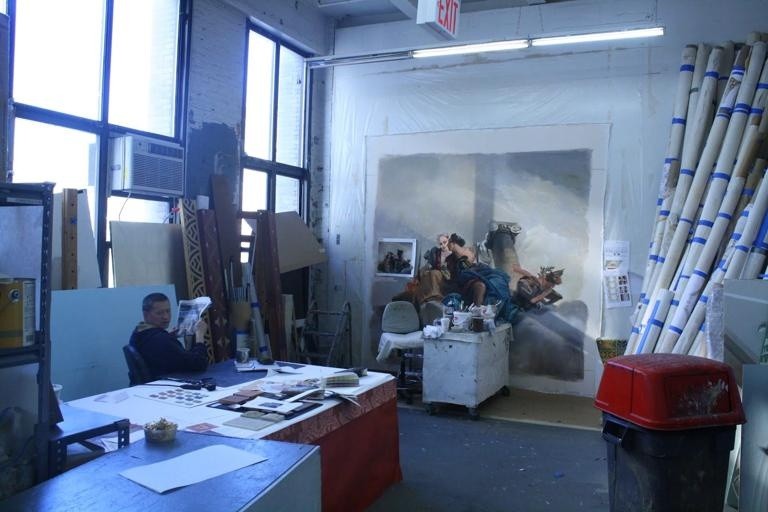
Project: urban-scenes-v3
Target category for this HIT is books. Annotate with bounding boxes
[218,365,367,431]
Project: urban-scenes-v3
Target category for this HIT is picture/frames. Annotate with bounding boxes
[374,238,421,277]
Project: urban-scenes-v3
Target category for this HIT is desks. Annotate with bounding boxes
[2,426,322,510]
[53,401,130,476]
[67,359,400,511]
[423,323,512,414]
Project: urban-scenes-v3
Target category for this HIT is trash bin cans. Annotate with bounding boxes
[593,353,747,512]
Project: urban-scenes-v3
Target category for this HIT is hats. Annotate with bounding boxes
[449,233,465,247]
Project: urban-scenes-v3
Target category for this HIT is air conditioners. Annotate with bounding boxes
[107,134,186,201]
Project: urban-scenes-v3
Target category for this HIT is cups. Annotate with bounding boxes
[441,318,450,332]
[237,347,250,363]
[473,317,483,332]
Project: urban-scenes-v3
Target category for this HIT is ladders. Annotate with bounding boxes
[294,300,352,366]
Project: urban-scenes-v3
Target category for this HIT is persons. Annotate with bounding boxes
[129,293,208,377]
[378,233,564,326]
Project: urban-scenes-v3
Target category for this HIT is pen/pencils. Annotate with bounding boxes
[495,299,504,311]
[460,300,477,312]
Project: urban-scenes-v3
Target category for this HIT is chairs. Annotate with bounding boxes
[123,345,152,389]
[377,300,454,405]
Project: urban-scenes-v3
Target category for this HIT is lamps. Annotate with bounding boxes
[406,0,667,60]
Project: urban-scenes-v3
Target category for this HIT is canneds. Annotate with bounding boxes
[471,316,484,332]
